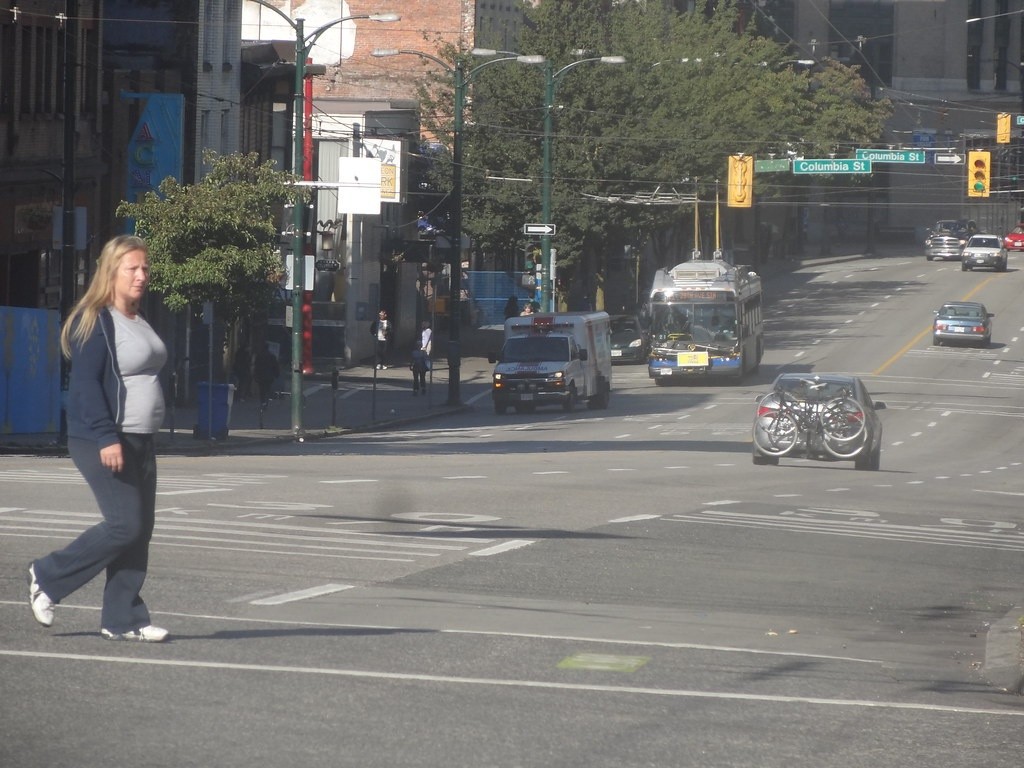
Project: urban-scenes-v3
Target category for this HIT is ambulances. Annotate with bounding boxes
[486,310,614,415]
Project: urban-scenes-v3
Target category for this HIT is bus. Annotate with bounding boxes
[646,257,765,385]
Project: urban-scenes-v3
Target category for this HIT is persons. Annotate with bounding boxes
[29,235,169,642]
[234,343,251,403]
[410,340,430,397]
[371,309,394,370]
[420,320,432,356]
[504,295,542,321]
[254,343,280,399]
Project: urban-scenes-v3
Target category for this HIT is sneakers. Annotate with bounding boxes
[100,626,170,644]
[27,561,55,627]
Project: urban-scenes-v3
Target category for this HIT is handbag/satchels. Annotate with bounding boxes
[425,357,431,371]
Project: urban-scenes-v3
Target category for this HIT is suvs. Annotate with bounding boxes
[923,218,979,262]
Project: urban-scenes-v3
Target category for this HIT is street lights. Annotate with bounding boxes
[247,0,404,445]
[470,46,629,313]
[369,47,547,406]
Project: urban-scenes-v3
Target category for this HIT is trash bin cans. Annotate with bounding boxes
[193,382,238,441]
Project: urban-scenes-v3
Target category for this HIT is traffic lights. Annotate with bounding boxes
[967,152,990,198]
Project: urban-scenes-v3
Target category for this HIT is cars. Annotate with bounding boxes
[961,234,1008,272]
[754,371,888,469]
[605,314,651,364]
[1004,222,1024,251]
[932,301,996,349]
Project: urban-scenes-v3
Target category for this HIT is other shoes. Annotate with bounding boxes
[421,389,426,394]
[383,365,388,369]
[376,364,382,370]
[413,389,418,394]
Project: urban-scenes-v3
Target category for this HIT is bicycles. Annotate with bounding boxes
[752,375,870,461]
